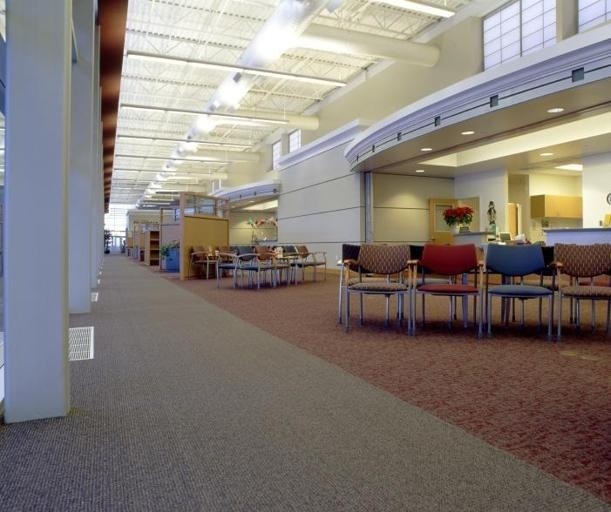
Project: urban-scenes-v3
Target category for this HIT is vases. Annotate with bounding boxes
[448,222,459,235]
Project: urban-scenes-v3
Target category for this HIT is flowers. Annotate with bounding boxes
[441,207,475,226]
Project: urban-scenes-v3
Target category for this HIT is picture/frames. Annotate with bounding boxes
[428,198,457,240]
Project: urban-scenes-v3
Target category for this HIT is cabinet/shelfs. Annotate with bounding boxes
[144,231,159,266]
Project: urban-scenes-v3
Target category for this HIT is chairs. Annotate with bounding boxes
[335,239,611,342]
[187,243,328,291]
